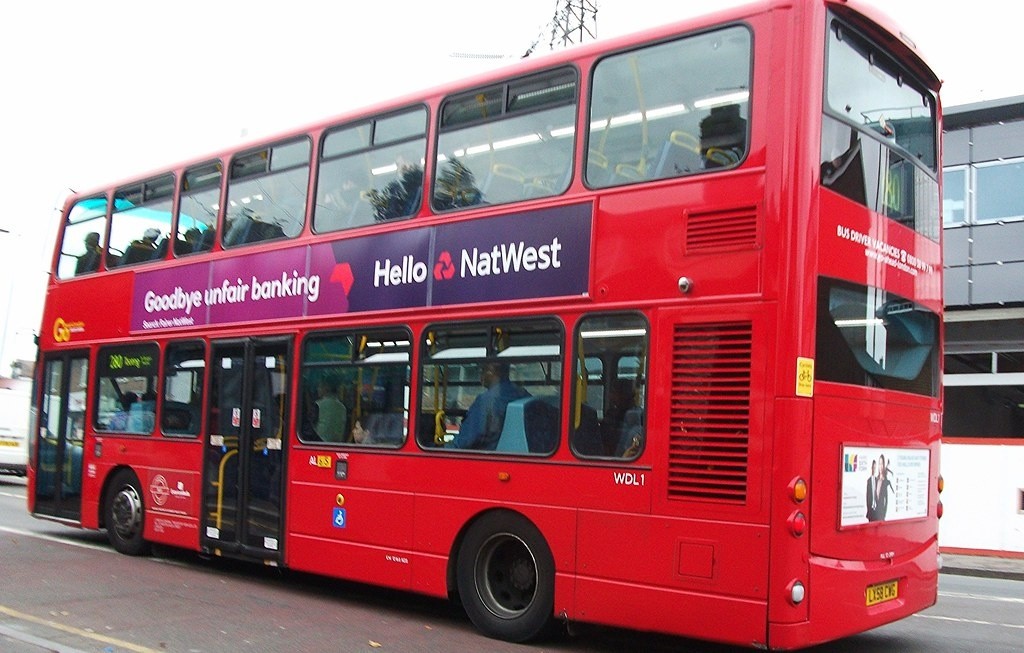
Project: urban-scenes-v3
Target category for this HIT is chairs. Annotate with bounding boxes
[81,128,744,455]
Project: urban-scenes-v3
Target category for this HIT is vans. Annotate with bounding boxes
[0,388,59,477]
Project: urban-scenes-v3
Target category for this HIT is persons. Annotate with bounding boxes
[442,351,530,450]
[866,454,893,520]
[106,392,156,432]
[76,208,260,270]
[600,377,644,454]
[305,376,349,442]
[352,416,377,445]
[324,153,425,220]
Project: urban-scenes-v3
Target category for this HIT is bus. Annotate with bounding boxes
[26,0,945,652]
